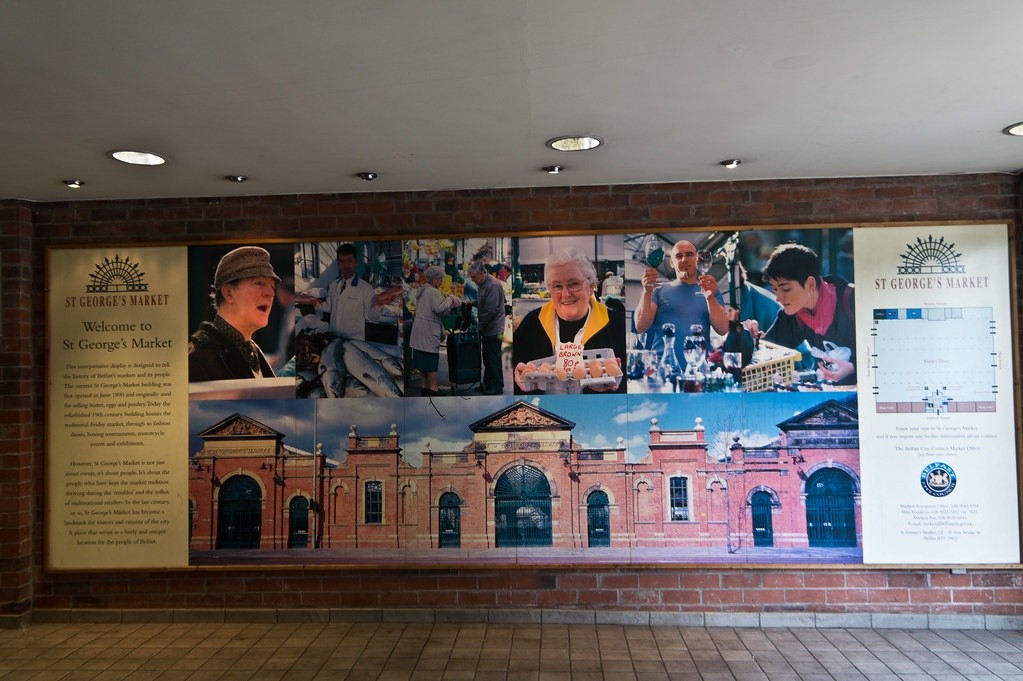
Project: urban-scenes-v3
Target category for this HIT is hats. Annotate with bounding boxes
[212,246,283,292]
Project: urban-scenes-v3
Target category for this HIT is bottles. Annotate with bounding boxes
[629,321,736,392]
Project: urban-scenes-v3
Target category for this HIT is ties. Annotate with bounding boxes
[338,280,347,295]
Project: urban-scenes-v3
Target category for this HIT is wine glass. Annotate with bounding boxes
[643,240,663,288]
[692,249,713,296]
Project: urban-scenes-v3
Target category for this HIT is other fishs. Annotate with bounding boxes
[297,336,403,397]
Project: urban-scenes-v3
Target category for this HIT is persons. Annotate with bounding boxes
[738,243,857,385]
[463,261,506,395]
[188,246,282,383]
[633,240,730,373]
[514,247,626,395]
[298,243,395,340]
[409,266,458,397]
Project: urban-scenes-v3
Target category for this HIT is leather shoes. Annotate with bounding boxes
[474,383,485,391]
[482,385,503,395]
[427,387,447,397]
[420,387,432,395]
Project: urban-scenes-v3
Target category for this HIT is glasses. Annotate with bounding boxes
[543,275,593,295]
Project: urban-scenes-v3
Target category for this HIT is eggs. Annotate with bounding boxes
[521,358,622,381]
